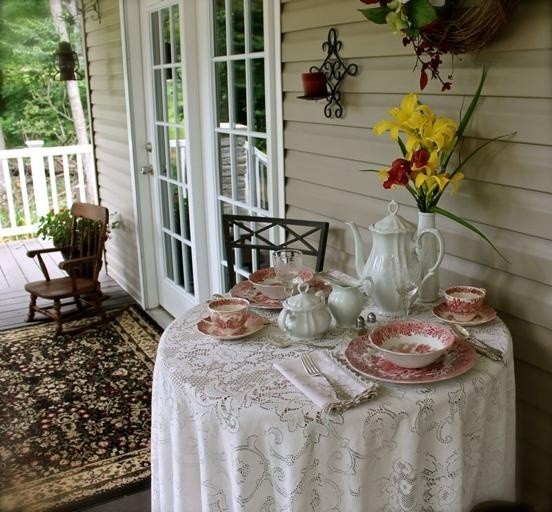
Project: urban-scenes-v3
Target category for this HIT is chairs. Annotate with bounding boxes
[22,200,110,338]
[220,213,330,289]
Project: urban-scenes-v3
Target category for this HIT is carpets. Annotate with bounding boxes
[0,302,165,511]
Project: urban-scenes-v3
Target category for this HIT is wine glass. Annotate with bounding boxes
[272,249,304,300]
[392,266,421,318]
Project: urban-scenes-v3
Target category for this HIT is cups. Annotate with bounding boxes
[205,295,250,336]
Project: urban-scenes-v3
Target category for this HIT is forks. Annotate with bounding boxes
[299,352,343,402]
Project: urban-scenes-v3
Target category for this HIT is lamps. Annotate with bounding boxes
[52,19,84,82]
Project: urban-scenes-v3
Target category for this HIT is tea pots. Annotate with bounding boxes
[327,276,374,329]
[344,199,443,316]
[277,283,332,342]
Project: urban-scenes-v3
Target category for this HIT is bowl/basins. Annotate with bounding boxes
[445,283,486,321]
[369,321,455,369]
[248,267,313,300]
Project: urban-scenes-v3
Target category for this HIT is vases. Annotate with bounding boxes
[412,210,442,303]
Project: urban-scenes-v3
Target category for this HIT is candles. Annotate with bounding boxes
[301,70,328,97]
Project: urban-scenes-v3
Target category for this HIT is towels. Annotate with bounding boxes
[272,347,378,417]
[313,268,366,294]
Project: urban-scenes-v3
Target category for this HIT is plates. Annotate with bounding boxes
[345,333,478,383]
[231,280,326,310]
[197,312,263,340]
[432,299,497,326]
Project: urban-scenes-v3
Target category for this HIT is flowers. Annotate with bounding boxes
[359,59,516,265]
